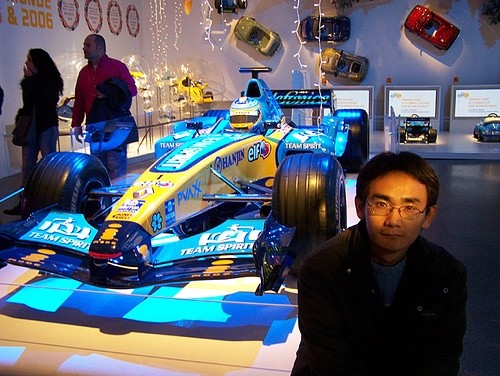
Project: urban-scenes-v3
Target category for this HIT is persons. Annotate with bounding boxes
[290,150,468,376]
[2,48,63,215]
[72,34,137,177]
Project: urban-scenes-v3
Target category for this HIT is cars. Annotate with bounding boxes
[318,47,370,83]
[55,96,76,121]
[404,5,460,51]
[233,15,281,57]
[299,14,351,41]
[214,0,248,15]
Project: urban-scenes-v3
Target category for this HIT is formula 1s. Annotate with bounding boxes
[399,114,437,144]
[473,112,500,143]
[0,66,370,296]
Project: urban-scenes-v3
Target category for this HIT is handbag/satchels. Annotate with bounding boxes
[12,108,33,145]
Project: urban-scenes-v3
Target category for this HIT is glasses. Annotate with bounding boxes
[366,199,428,220]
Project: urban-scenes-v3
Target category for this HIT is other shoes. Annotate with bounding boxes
[4,205,21,216]
